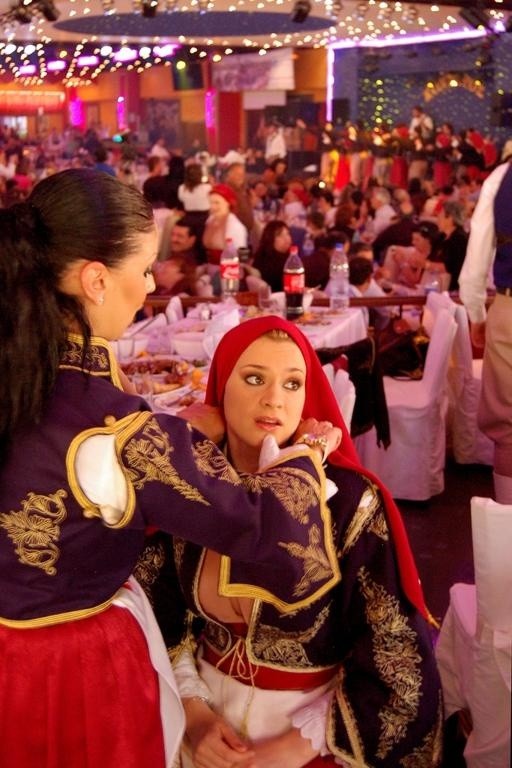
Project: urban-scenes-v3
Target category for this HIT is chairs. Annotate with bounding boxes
[448,304,496,466]
[419,291,458,337]
[354,307,457,501]
[434,495,512,768]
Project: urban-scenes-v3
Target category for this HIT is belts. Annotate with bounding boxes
[495,285,512,297]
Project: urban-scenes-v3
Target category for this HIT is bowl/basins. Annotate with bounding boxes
[170,330,209,361]
[108,331,149,358]
[293,319,334,337]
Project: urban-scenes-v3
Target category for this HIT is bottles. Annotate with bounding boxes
[282,244,306,321]
[219,238,241,304]
[328,243,351,314]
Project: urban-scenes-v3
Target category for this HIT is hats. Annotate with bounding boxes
[442,200,466,226]
[208,182,240,214]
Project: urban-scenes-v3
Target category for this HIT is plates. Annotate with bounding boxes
[125,362,195,404]
